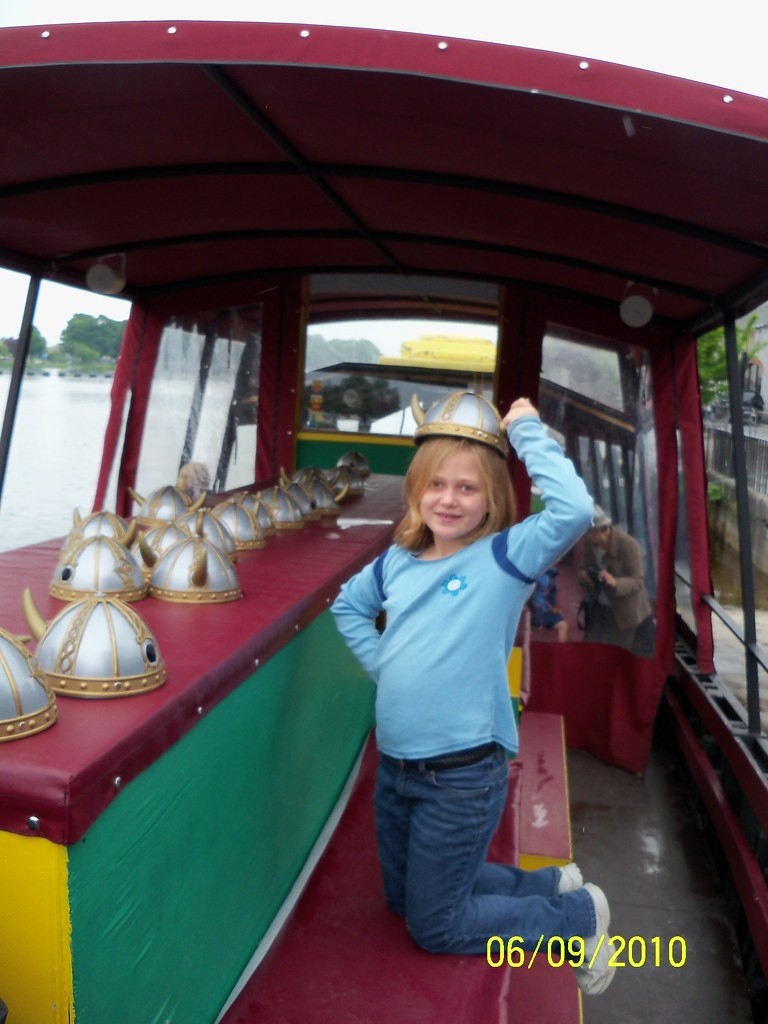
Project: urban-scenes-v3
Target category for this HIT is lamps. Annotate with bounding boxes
[87,253,126,296]
[619,280,660,328]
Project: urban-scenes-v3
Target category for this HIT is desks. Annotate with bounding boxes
[0,471,410,1024]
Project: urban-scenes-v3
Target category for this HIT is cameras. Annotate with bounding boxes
[587,566,606,589]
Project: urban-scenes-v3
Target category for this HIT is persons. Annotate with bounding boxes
[523,562,569,648]
[578,503,653,652]
[327,390,620,997]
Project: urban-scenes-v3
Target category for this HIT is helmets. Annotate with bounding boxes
[336,448,372,478]
[278,466,350,521]
[148,536,242,604]
[224,491,276,539]
[327,464,365,497]
[210,498,266,552]
[410,392,516,458]
[48,518,148,602]
[174,507,237,562]
[125,486,207,530]
[60,506,136,557]
[134,522,192,587]
[251,484,305,530]
[0,628,63,742]
[584,505,612,530]
[22,586,169,699]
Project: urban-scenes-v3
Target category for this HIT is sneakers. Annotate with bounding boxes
[559,861,583,892]
[574,881,618,997]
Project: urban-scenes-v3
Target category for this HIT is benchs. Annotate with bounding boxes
[218,713,584,1024]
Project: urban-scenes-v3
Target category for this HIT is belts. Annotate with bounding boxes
[379,741,497,774]
